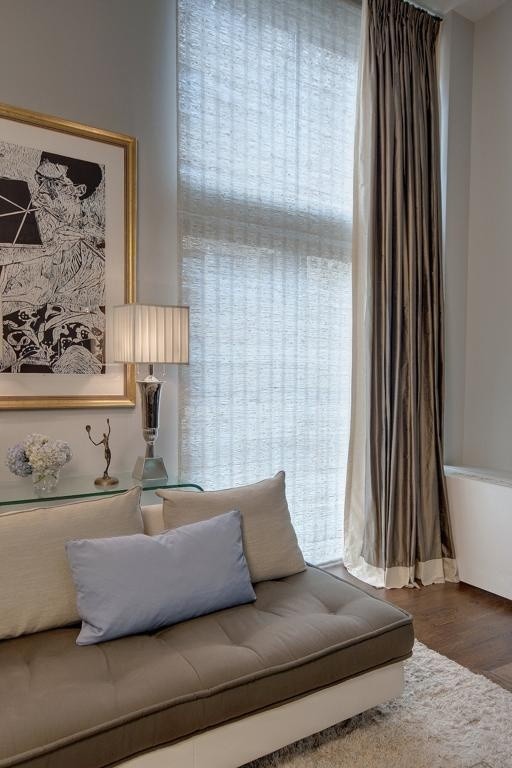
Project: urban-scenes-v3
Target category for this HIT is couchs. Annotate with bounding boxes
[0,565,413,768]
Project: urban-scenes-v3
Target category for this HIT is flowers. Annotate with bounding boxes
[8,431,72,482]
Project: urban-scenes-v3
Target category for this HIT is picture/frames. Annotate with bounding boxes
[0,102,139,411]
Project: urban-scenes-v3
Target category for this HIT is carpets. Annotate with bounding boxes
[242,633,512,767]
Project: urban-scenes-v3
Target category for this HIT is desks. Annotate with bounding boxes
[0,473,204,512]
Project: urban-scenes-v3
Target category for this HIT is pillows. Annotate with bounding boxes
[2,484,144,637]
[62,511,254,648]
[154,469,305,583]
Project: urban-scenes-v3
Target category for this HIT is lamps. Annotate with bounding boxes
[114,304,190,483]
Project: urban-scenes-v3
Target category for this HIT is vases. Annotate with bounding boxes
[31,469,62,493]
